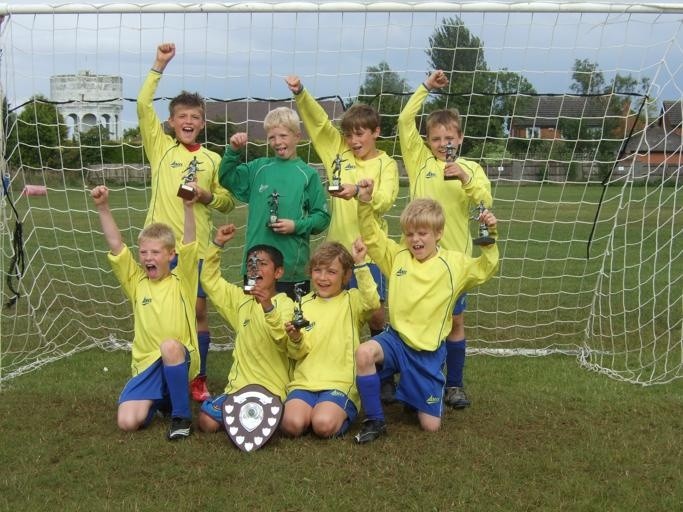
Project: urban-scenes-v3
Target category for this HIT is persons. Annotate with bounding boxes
[181,162,206,180]
[218,107,330,300]
[181,155,207,180]
[439,138,459,165]
[329,152,348,186]
[200,224,295,440]
[354,180,501,442]
[136,42,234,403]
[87,184,203,438]
[465,198,486,227]
[398,66,495,411]
[282,73,399,334]
[278,239,383,439]
[289,280,312,324]
[245,248,262,281]
[267,187,285,217]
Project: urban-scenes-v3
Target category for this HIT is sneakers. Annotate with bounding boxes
[379,380,395,403]
[443,386,470,409]
[188,380,209,401]
[353,422,384,444]
[169,418,191,439]
